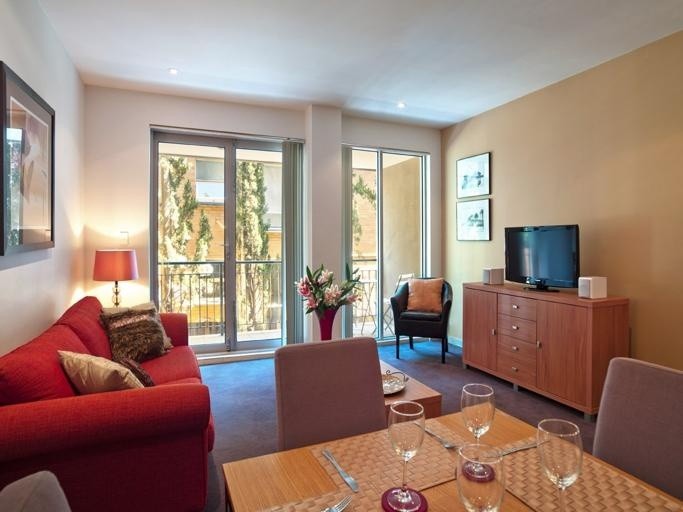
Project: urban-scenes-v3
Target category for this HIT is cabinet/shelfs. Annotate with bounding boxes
[462,281,630,422]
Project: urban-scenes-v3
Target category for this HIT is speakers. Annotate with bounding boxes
[483,267,504,284]
[578,275,607,299]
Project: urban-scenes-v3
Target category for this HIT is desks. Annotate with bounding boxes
[352,278,377,335]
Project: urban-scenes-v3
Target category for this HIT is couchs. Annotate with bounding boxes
[0,296,214,512]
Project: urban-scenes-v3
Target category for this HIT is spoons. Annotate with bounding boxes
[413,420,458,449]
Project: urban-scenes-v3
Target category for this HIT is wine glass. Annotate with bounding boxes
[388,401,425,512]
[535,420,580,512]
[462,383,494,461]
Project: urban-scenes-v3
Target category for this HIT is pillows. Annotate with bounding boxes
[55,347,154,394]
[406,277,444,313]
[98,299,174,362]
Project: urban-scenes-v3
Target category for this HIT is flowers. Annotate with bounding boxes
[292,263,365,321]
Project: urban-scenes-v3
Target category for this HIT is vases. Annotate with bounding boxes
[314,309,336,340]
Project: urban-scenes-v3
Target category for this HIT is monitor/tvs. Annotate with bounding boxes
[504,224,580,293]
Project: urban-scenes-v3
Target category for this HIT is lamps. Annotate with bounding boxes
[92,248,140,308]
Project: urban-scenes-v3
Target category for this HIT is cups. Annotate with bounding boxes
[455,446,504,512]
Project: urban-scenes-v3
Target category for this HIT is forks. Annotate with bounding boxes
[324,494,354,511]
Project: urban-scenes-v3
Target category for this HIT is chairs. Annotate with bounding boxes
[389,277,452,364]
[370,273,414,336]
[594,356,683,503]
[273,336,388,451]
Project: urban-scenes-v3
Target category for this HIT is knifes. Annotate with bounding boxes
[321,449,359,491]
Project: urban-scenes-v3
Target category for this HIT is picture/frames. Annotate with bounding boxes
[454,152,491,241]
[0,61,55,258]
